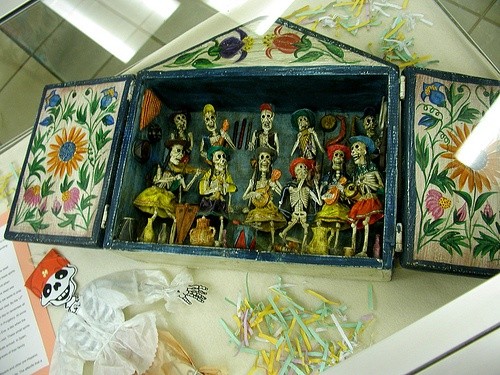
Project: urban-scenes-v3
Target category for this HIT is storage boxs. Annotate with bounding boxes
[3,64,499,280]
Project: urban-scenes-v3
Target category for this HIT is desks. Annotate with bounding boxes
[0,0,498,375]
